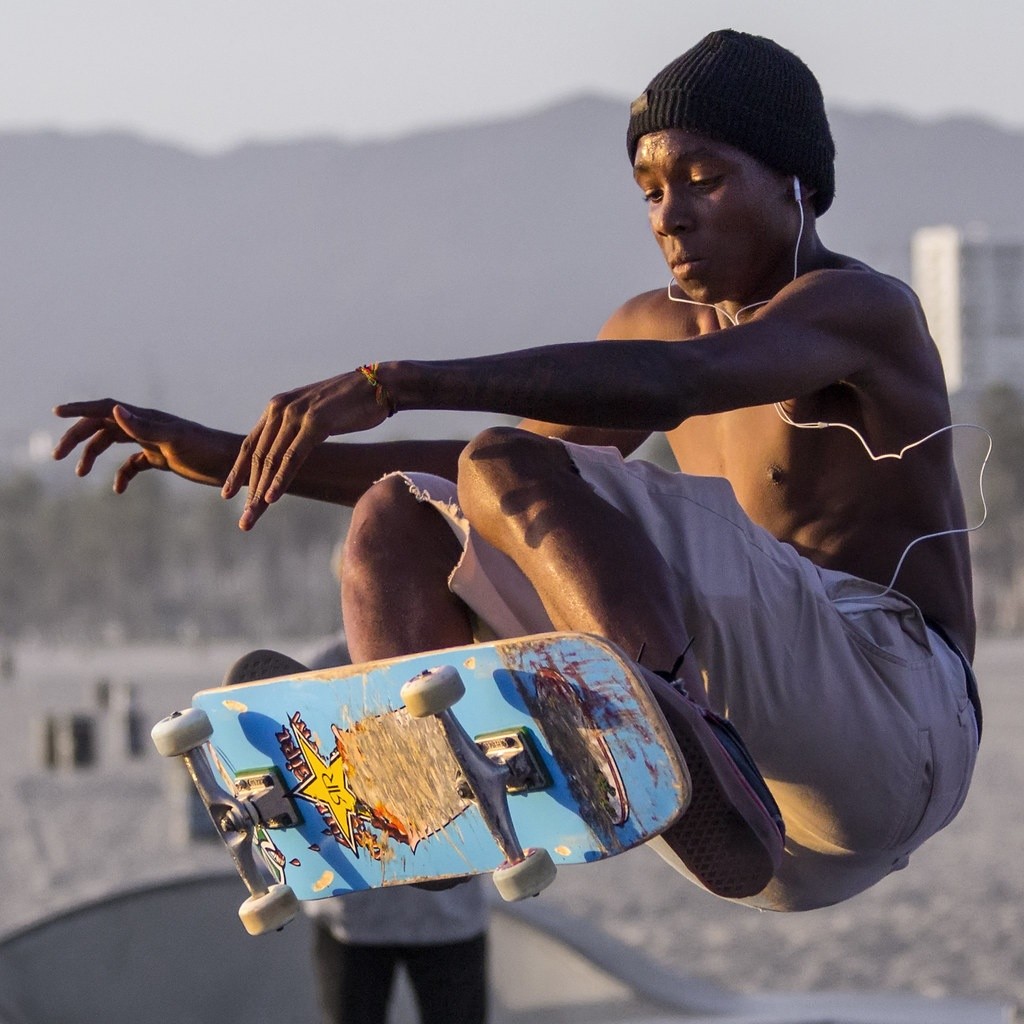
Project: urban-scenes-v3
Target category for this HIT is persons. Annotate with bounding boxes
[295,637,496,1024]
[56,25,984,1024]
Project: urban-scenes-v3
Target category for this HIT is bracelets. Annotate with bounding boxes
[357,363,399,422]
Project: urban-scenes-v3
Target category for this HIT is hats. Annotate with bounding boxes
[627,29,836,218]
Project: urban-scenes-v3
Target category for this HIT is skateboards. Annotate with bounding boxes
[148,629,696,938]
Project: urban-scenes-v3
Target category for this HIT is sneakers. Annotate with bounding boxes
[633,659,787,899]
[222,650,310,689]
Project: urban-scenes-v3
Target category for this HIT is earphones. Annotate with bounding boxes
[792,175,802,202]
